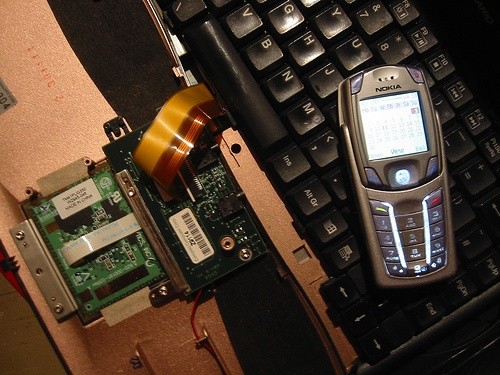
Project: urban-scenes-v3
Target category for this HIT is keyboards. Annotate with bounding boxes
[145,0,500,375]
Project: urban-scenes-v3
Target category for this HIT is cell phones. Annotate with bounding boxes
[338,64,458,288]
